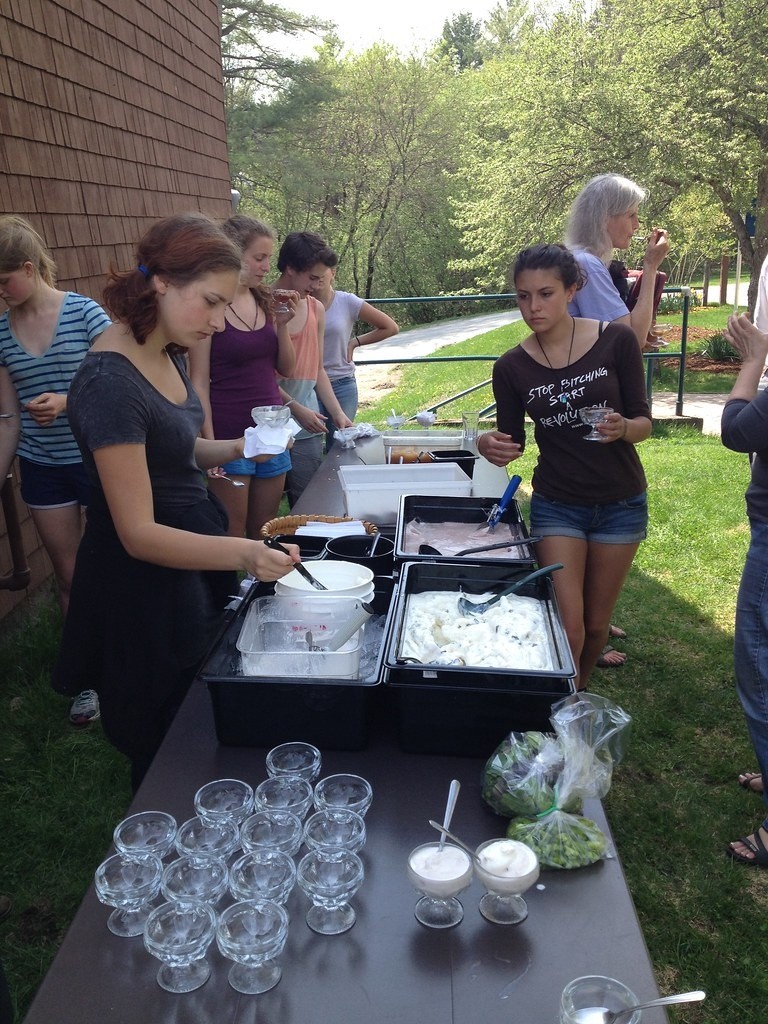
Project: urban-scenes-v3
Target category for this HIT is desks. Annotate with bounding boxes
[30,427,671,1024]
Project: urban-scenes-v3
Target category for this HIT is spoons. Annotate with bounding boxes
[569,991,705,1024]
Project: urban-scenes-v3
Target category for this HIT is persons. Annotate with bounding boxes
[720,253,768,866]
[0,216,114,724]
[186,213,301,540]
[560,173,670,669]
[310,252,399,458]
[66,215,300,797]
[268,230,353,511]
[476,242,653,698]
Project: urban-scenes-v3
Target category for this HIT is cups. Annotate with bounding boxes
[462,412,479,439]
[560,974,643,1024]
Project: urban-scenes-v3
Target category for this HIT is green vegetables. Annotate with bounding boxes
[482,730,584,819]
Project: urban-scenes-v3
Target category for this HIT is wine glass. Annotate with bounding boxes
[272,289,295,313]
[337,427,359,449]
[406,842,473,929]
[580,407,615,441]
[416,414,435,430]
[650,323,673,347]
[473,838,540,926]
[387,416,404,430]
[93,741,373,994]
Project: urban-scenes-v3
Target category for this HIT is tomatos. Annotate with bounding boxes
[384,449,431,463]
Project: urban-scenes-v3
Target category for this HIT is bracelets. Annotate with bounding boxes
[621,417,627,440]
[477,435,484,456]
[356,336,361,346]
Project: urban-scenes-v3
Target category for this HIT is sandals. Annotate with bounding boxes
[725,828,768,866]
[739,775,764,794]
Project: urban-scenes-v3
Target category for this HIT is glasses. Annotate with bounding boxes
[621,266,626,271]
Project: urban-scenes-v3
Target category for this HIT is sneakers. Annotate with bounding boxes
[69,689,101,724]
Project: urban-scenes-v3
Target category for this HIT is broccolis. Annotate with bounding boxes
[509,815,606,870]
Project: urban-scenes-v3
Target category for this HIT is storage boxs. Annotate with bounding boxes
[381,429,464,465]
[336,460,473,523]
[236,595,366,681]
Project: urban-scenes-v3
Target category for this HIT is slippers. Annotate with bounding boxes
[597,646,628,666]
[608,622,626,638]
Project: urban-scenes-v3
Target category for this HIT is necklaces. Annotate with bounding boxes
[536,316,576,404]
[228,301,259,334]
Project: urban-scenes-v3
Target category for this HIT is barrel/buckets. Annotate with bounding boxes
[277,559,374,650]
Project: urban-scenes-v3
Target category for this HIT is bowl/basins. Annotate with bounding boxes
[251,405,291,428]
[326,535,395,573]
